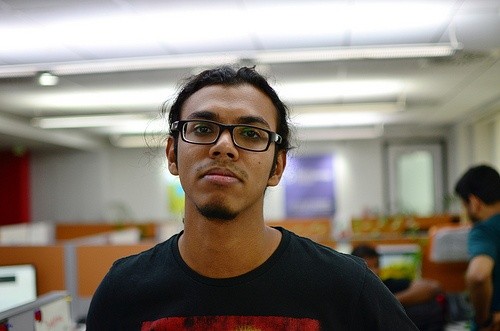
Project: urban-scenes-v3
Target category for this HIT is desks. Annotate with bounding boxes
[1,217,499,330]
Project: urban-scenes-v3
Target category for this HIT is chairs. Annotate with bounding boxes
[0,264,35,313]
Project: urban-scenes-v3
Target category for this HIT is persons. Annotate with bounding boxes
[85,64,417,331]
[455,164,500,331]
[350,244,438,305]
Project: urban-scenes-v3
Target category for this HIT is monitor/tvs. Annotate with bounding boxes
[0,264,37,312]
[377,243,423,281]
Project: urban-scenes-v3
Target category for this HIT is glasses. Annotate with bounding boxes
[170,119,283,152]
